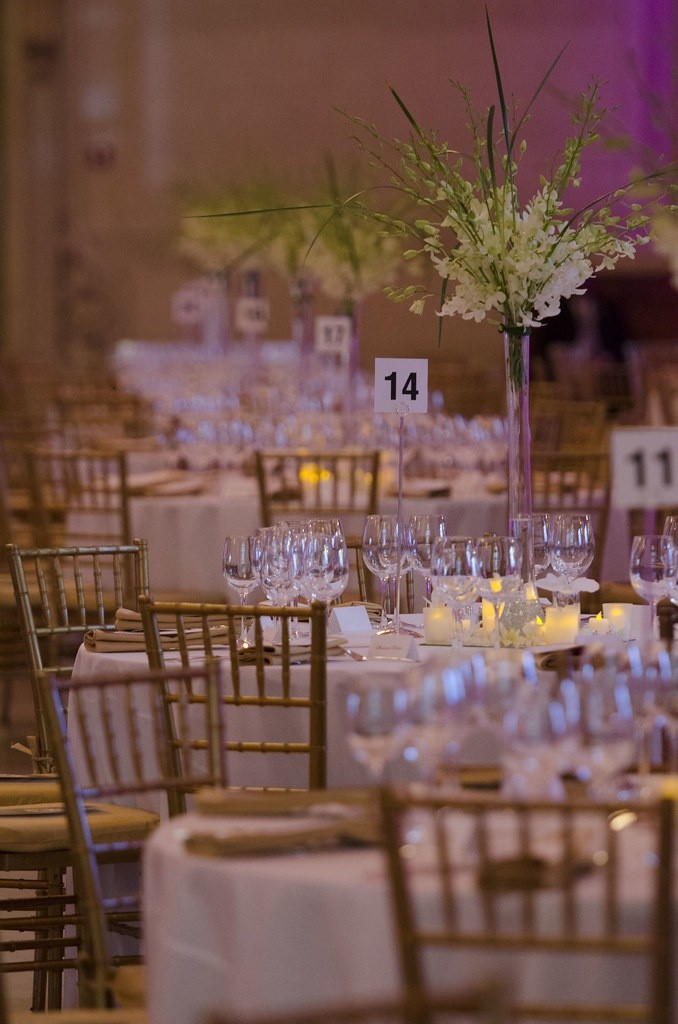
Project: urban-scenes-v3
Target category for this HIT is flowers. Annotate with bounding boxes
[185,3,678,402]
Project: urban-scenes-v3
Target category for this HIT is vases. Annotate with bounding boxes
[502,330,535,576]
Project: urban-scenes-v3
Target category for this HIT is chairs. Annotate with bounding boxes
[0,423,678,1024]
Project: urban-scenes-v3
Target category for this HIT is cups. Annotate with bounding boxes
[509,511,598,609]
[661,515,678,607]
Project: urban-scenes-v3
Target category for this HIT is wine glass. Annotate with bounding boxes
[359,515,424,640]
[107,333,515,484]
[339,640,678,845]
[220,535,262,656]
[251,518,349,653]
[407,512,448,606]
[629,535,678,645]
[429,533,524,661]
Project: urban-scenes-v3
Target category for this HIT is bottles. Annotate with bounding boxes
[534,468,603,502]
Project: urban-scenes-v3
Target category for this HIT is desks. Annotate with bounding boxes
[53,611,666,975]
[65,463,510,607]
